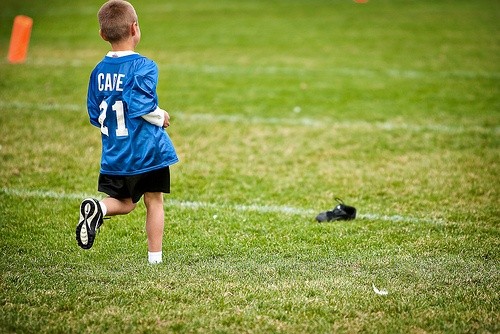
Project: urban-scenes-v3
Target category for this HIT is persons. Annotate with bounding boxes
[76,0,179,264]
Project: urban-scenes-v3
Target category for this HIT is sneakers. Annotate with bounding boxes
[76,199,103,250]
[316,198,357,222]
